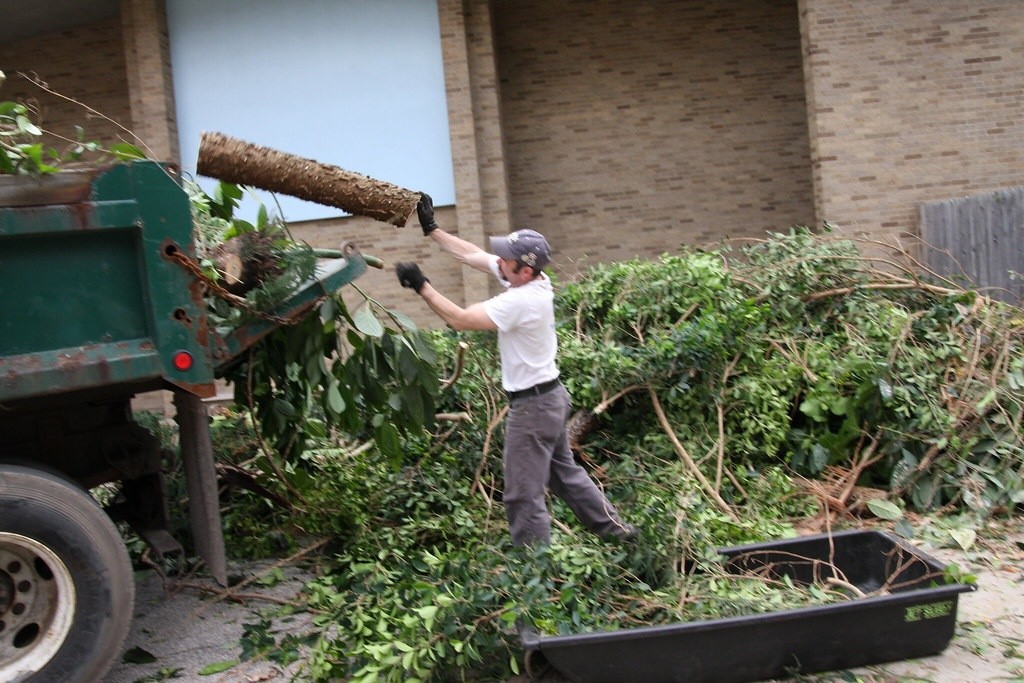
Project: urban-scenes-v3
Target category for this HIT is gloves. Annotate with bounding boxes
[393,261,430,293]
[417,191,439,236]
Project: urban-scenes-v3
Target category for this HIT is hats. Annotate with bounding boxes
[488,229,552,270]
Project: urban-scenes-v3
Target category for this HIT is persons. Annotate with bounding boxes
[396,192,638,550]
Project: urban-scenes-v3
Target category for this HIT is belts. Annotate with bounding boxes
[500,377,560,401]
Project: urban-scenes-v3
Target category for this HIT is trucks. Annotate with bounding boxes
[0,161,369,683]
